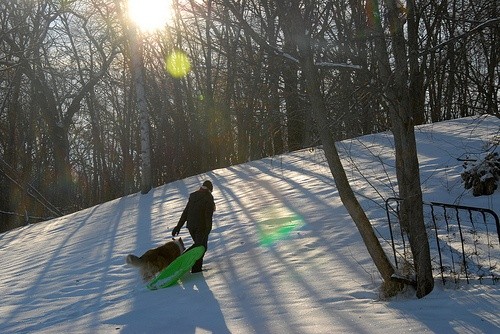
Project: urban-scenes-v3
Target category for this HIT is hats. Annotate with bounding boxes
[203,181,213,192]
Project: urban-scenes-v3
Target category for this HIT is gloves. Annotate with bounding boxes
[172,227,180,236]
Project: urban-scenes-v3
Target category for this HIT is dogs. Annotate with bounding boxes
[126,236,186,283]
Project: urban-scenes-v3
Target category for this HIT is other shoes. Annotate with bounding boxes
[192,266,202,273]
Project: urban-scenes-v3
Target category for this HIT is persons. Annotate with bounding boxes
[173,179,216,272]
